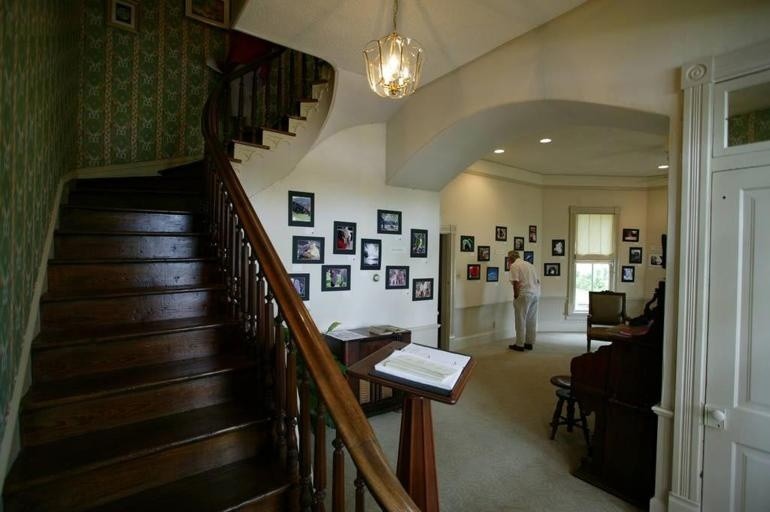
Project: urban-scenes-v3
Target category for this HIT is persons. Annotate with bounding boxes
[504,250,542,353]
[215,27,273,146]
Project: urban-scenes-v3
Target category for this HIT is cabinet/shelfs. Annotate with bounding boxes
[320,325,411,419]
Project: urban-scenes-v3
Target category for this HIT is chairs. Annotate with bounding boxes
[549,347,613,448]
[587,290,633,352]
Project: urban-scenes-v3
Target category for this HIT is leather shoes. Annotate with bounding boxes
[524,342,533,351]
[509,344,524,352]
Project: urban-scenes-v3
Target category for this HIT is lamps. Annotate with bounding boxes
[359,2,428,99]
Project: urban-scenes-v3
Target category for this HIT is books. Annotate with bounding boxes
[616,324,652,336]
[373,343,472,390]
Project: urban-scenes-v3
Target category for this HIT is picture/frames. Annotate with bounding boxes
[621,228,642,282]
[274,189,434,301]
[544,239,565,276]
[459,224,537,284]
